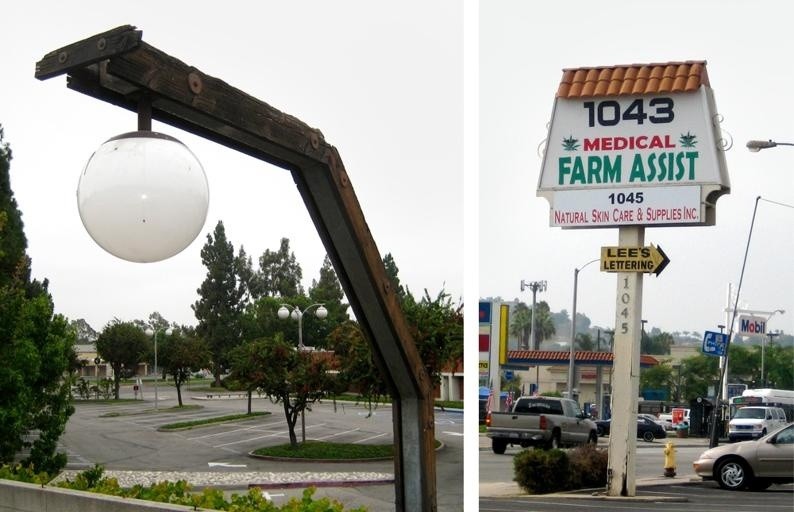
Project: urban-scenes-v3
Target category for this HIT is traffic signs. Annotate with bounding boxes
[600,243,670,277]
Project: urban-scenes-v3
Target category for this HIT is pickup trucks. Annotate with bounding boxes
[484,395,598,454]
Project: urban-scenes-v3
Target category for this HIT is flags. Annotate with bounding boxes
[486,381,496,415]
[505,386,513,408]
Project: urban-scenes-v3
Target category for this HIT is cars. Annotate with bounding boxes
[692,407,794,491]
[593,409,691,442]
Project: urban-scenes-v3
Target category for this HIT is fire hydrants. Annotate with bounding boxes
[664,440,678,477]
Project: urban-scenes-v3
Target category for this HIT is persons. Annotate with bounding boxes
[591,400,597,417]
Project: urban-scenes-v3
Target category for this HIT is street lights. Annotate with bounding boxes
[143,324,174,412]
[751,310,786,388]
[34,23,437,512]
[746,139,794,152]
[521,279,547,351]
[277,303,328,448]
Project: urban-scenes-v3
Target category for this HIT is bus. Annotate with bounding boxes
[727,388,794,422]
